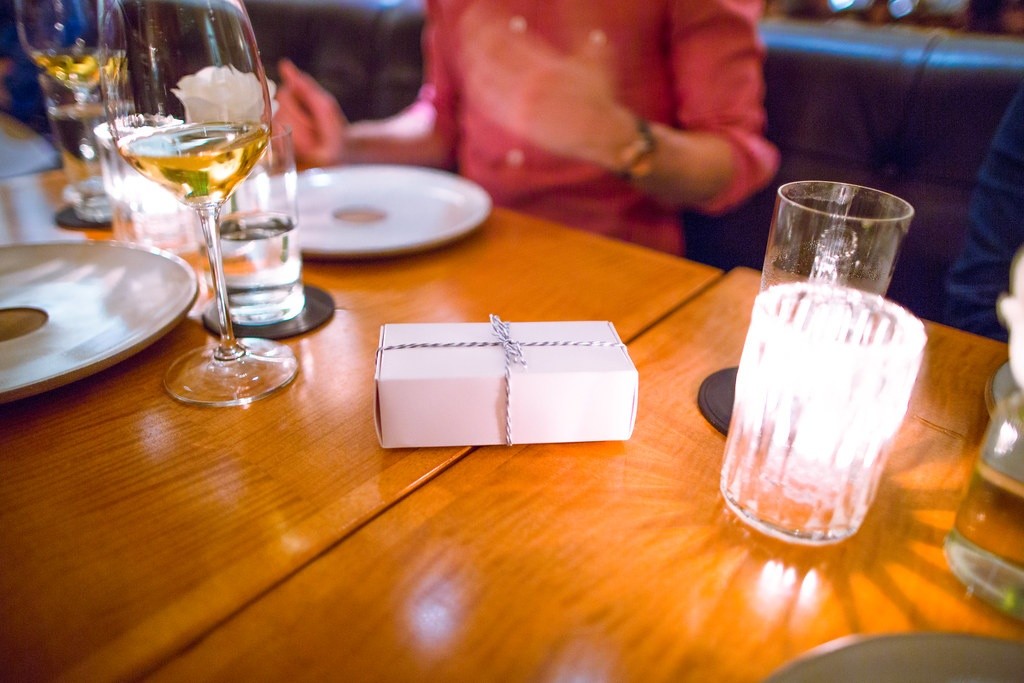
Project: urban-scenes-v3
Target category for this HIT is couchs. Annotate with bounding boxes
[263,0,1024,328]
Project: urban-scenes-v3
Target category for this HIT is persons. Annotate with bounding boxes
[271,0,781,254]
[939,77,1024,343]
[0,0,94,134]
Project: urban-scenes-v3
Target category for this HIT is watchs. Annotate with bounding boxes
[620,117,658,180]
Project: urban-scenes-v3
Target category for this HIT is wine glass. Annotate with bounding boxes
[14,0,127,223]
[97,0,299,408]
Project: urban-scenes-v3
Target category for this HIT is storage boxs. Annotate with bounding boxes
[375,319,639,447]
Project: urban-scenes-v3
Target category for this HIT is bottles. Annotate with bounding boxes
[946,388,1023,625]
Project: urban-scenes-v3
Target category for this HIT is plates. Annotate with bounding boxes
[219,164,494,259]
[765,628,1024,683]
[0,240,199,405]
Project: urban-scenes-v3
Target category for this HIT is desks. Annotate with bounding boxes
[0,108,1024,683]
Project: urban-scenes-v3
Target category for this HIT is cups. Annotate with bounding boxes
[35,72,137,223]
[759,179,915,299]
[719,281,928,547]
[193,121,305,327]
